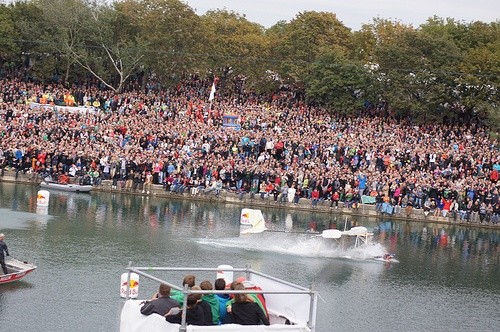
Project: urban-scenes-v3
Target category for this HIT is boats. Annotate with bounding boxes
[0,252,37,284]
[119,261,318,332]
[240,207,395,262]
[40,175,93,193]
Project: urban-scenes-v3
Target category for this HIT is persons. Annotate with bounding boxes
[200,281,220,324]
[171,274,195,307]
[221,284,270,325]
[140,284,179,316]
[213,278,231,324]
[165,286,212,326]
[0,75,500,223]
[0,234,9,275]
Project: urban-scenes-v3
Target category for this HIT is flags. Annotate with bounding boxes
[209,83,216,101]
